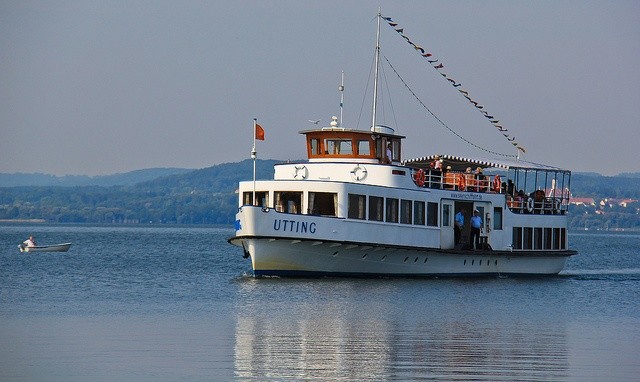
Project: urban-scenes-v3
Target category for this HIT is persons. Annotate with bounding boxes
[23,236,37,247]
[502,178,529,201]
[425,156,489,190]
[387,143,393,161]
[470,210,483,250]
[454,209,466,246]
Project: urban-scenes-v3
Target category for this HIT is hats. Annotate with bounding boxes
[475,209,479,213]
[446,166,451,170]
[439,159,444,163]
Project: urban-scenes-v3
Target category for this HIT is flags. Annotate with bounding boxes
[382,15,527,154]
[256,124,264,140]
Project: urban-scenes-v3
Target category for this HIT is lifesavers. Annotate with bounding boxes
[527,198,534,212]
[494,177,500,190]
[416,169,424,186]
[458,174,465,190]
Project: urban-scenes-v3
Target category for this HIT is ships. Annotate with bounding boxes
[226,5,579,281]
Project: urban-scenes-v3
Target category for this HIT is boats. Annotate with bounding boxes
[17,241,72,254]
